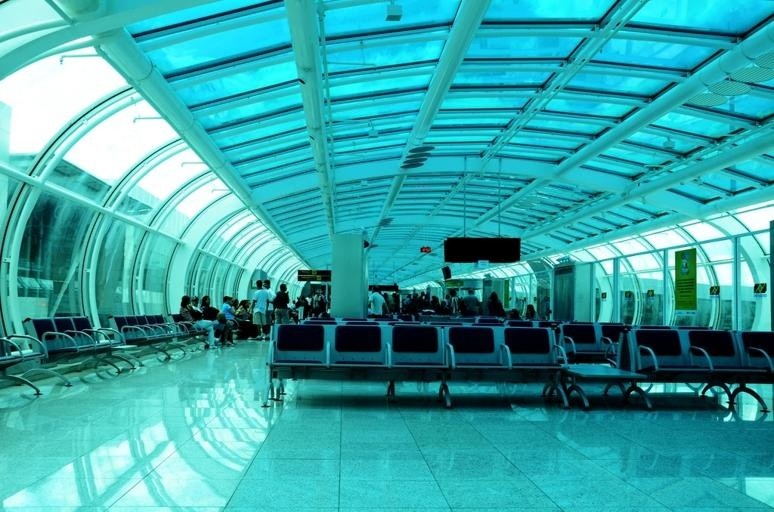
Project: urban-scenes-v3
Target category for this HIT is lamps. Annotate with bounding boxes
[386,0,402,21]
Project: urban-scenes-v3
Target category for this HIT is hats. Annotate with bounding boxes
[191,295,200,300]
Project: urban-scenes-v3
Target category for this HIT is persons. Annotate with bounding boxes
[526,304,539,321]
[509,308,523,320]
[488,292,507,316]
[385,290,430,313]
[368,306,382,315]
[190,296,205,319]
[431,296,439,306]
[435,301,452,314]
[449,289,459,314]
[263,280,277,340]
[180,296,219,348]
[287,287,329,324]
[232,298,252,323]
[268,284,290,325]
[247,280,270,340]
[222,296,240,340]
[368,285,389,317]
[200,296,232,345]
[460,289,480,315]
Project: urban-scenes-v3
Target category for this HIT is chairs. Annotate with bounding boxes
[458,325,512,408]
[22,316,134,386]
[513,325,569,407]
[111,315,189,366]
[629,329,774,412]
[263,325,331,408]
[0,334,71,396]
[558,322,643,357]
[394,325,457,408]
[172,314,211,349]
[332,326,393,407]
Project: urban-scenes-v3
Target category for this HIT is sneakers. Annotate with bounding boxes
[209,345,218,349]
[212,319,219,325]
[265,336,270,340]
[247,336,257,339]
[224,340,232,344]
[256,335,261,340]
